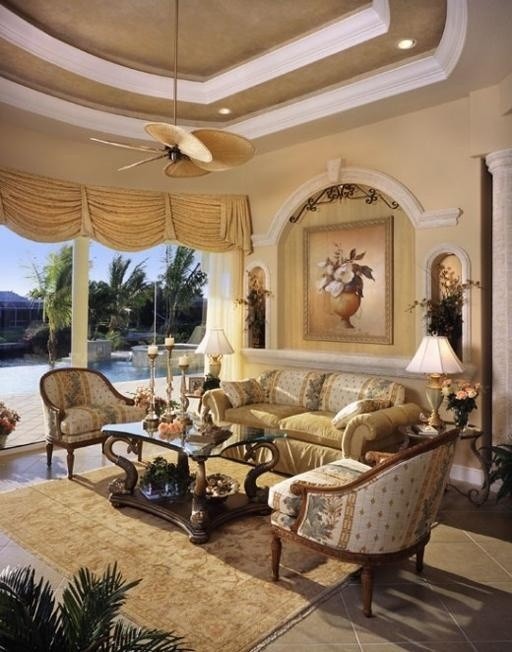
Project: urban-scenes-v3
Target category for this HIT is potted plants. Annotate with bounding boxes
[404,261,481,357]
[230,270,271,348]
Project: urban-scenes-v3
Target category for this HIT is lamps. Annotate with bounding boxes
[195,328,235,383]
[405,330,466,427]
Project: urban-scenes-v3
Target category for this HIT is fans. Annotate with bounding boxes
[88,0,255,178]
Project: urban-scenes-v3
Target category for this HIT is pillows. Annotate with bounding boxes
[331,399,391,429]
[219,378,265,409]
[257,368,333,411]
[318,372,407,414]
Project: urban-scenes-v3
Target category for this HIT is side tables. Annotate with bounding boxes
[398,420,490,506]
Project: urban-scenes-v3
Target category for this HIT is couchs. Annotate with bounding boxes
[203,367,422,475]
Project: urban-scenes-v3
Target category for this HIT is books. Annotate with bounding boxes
[184,423,233,443]
[410,423,439,435]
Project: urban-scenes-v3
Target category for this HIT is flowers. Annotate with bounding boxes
[440,378,480,426]
[0,401,21,434]
[315,240,375,298]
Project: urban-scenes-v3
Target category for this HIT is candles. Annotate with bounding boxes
[148,343,158,354]
[164,333,175,346]
[179,354,189,365]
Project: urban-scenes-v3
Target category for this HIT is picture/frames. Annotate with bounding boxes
[302,215,394,345]
[189,377,205,394]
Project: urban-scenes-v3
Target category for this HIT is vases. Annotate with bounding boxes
[0,433,11,449]
[454,408,471,434]
[330,288,360,328]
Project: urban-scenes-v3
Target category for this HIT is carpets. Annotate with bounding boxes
[0,447,445,652]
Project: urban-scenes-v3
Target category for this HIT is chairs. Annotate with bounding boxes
[40,368,147,480]
[267,427,460,618]
